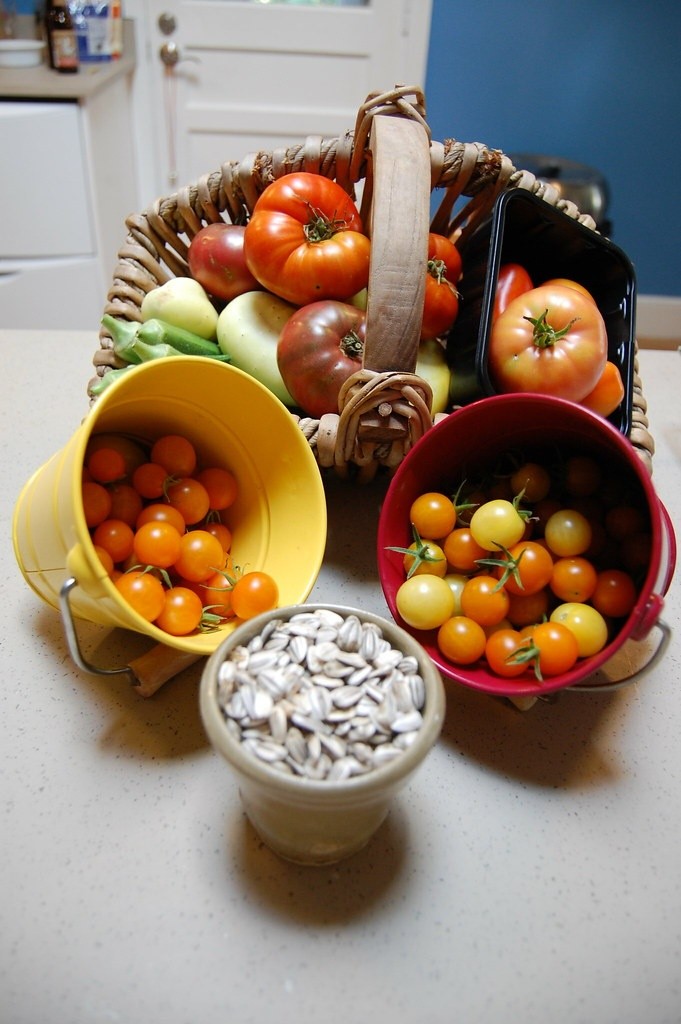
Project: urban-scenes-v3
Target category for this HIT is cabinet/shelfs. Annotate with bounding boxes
[0,72,137,331]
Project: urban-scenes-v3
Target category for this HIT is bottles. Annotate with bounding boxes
[68,0,124,61]
[45,0,79,74]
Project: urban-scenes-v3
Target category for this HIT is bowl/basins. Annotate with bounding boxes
[0,38,47,70]
[200,601,447,869]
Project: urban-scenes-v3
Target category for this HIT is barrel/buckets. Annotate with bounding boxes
[376,389,678,713]
[13,353,330,699]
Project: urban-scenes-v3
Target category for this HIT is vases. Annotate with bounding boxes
[200,602,446,869]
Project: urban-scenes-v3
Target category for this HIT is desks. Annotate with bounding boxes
[1,327,681,1022]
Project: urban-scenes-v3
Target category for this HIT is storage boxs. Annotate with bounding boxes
[455,190,639,439]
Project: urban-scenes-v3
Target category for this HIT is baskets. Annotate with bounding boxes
[88,80,655,484]
